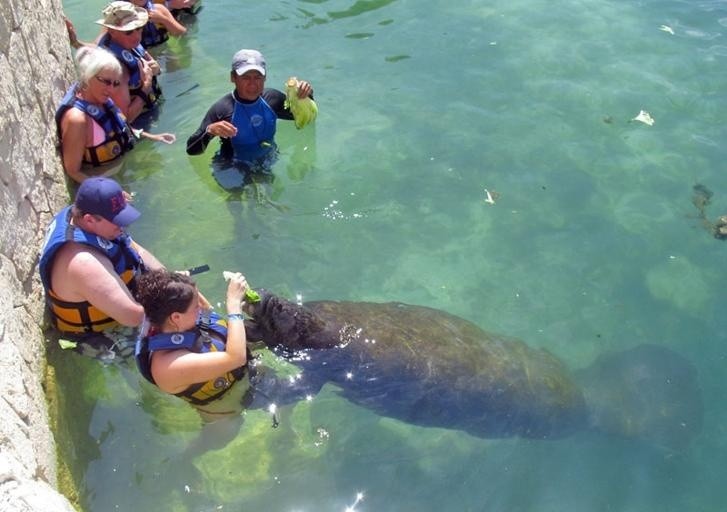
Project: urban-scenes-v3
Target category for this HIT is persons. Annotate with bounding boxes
[133,266,255,481]
[64,0,199,140]
[37,176,192,366]
[184,47,315,246]
[54,47,177,204]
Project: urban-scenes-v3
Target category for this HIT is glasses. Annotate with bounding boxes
[97,75,121,87]
[123,28,141,36]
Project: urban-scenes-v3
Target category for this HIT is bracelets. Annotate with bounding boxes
[229,310,245,322]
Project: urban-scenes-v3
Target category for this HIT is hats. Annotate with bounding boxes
[233,49,266,77]
[76,178,141,226]
[94,1,149,31]
